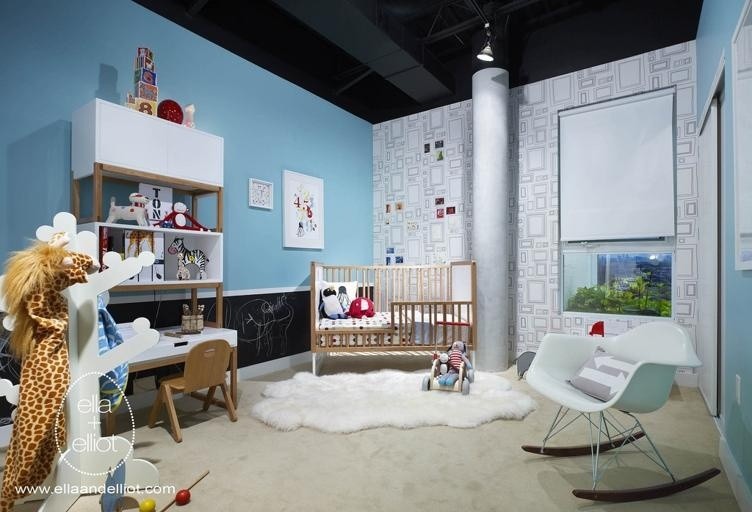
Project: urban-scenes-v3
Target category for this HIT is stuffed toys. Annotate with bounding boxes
[438,341,472,386]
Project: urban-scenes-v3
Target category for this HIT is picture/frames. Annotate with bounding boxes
[280,168,325,251]
[727,1,751,272]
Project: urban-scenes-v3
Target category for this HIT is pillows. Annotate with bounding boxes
[564,345,642,404]
[323,280,358,307]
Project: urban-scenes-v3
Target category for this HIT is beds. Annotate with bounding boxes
[309,261,478,376]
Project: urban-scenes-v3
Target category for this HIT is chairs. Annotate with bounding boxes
[523,321,723,505]
[148,339,238,442]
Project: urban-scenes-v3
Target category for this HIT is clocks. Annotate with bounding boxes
[248,177,274,210]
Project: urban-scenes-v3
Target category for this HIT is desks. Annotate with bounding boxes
[102,324,241,436]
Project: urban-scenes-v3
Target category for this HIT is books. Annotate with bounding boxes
[164,329,202,339]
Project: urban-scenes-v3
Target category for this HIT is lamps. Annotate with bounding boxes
[475,36,495,63]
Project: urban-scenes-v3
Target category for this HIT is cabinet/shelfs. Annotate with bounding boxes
[70,95,224,190]
[69,162,225,329]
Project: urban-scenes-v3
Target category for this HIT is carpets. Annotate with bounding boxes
[248,368,538,431]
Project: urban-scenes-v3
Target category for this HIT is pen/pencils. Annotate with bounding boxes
[182,304,205,316]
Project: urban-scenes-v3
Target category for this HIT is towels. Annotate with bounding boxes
[99,294,129,414]
